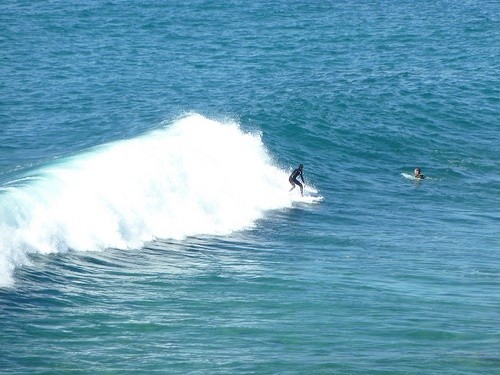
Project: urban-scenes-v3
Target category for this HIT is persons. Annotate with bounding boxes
[289,164,305,197]
[414,167,424,179]
